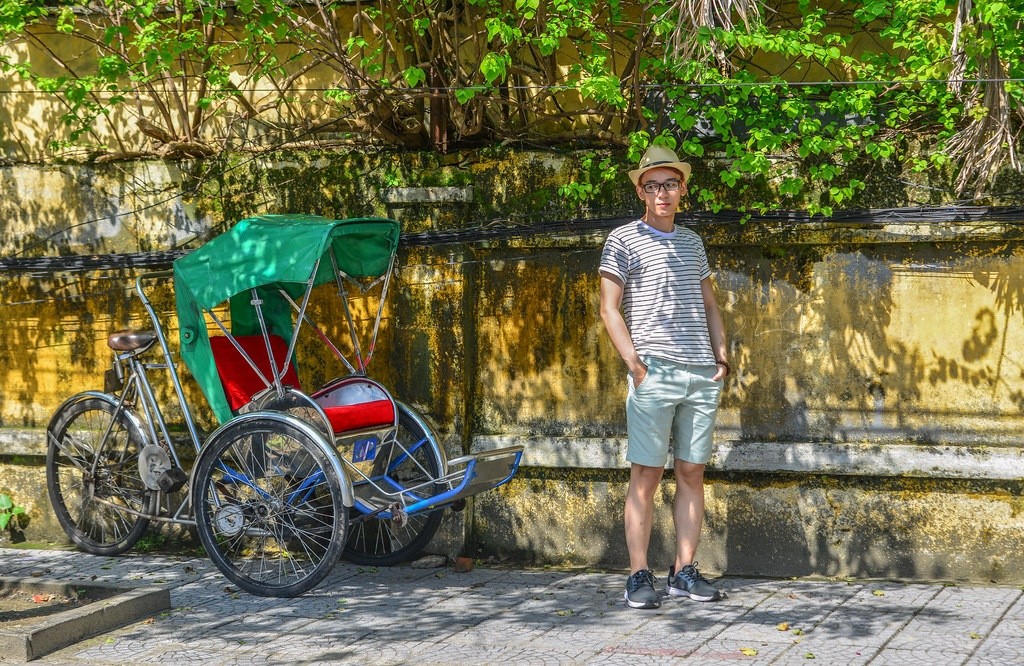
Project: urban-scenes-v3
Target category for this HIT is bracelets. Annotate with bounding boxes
[716,360,731,377]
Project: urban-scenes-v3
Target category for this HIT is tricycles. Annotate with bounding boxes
[46,215,523,598]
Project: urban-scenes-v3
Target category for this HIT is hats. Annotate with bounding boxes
[628,144,692,187]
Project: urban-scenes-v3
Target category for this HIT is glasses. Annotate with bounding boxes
[641,178,682,193]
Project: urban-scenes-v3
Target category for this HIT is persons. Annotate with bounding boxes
[596,145,729,610]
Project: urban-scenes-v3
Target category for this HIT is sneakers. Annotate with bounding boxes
[665,562,721,603]
[624,569,660,610]
